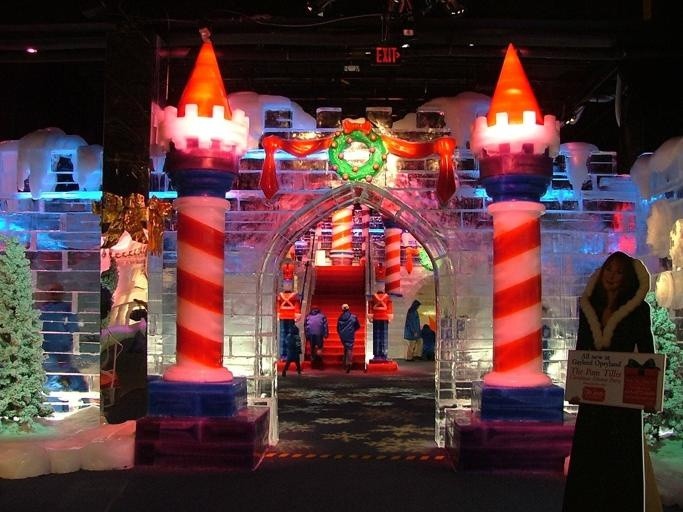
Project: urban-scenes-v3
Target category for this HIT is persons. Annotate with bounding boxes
[281,320,302,377]
[40,283,89,409]
[420,324,435,361]
[276,281,302,362]
[404,300,424,361]
[281,257,295,292]
[304,305,329,371]
[367,283,394,363]
[561,251,664,512]
[337,303,360,374]
[108,229,149,327]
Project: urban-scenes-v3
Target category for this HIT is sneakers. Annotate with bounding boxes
[282,365,288,376]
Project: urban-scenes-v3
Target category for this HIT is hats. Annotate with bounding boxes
[342,304,349,310]
